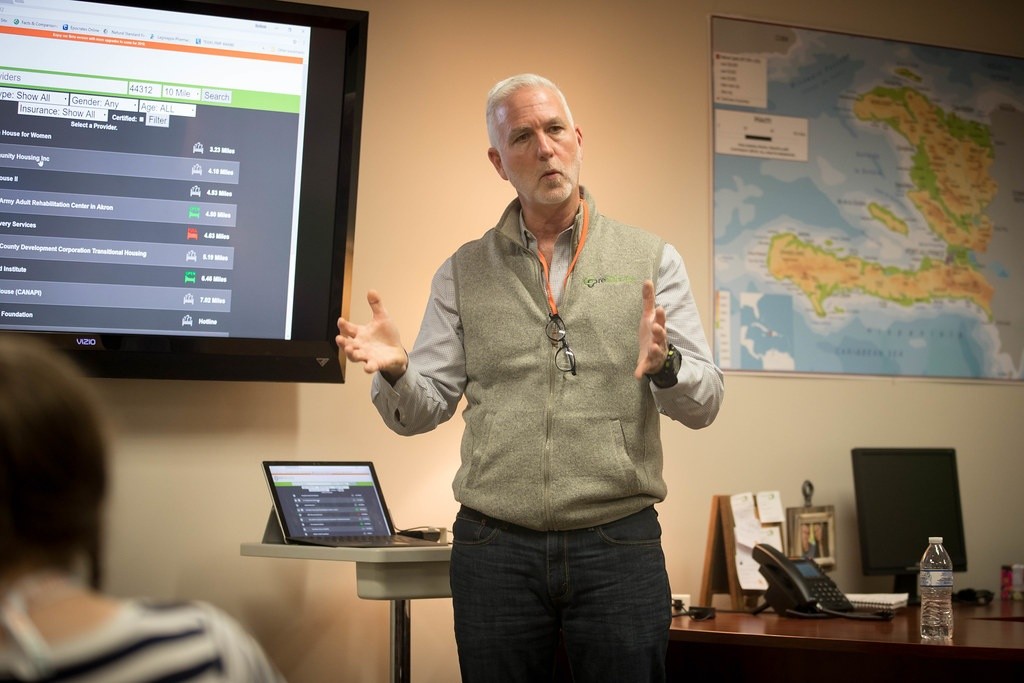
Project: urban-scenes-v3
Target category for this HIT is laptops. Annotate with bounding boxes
[260,459,449,547]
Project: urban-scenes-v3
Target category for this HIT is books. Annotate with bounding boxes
[844,592,909,609]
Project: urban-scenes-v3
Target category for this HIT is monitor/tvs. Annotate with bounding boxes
[850,446,967,577]
[0,0,371,383]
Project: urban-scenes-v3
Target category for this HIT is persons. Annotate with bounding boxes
[334,72,724,683]
[0,335,288,683]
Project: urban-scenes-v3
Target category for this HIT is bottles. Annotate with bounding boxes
[918,537,954,641]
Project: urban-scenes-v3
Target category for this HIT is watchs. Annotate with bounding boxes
[643,342,682,381]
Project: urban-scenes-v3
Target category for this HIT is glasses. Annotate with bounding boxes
[547,314,576,377]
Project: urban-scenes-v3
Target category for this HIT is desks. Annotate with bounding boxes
[663,600,1024,683]
